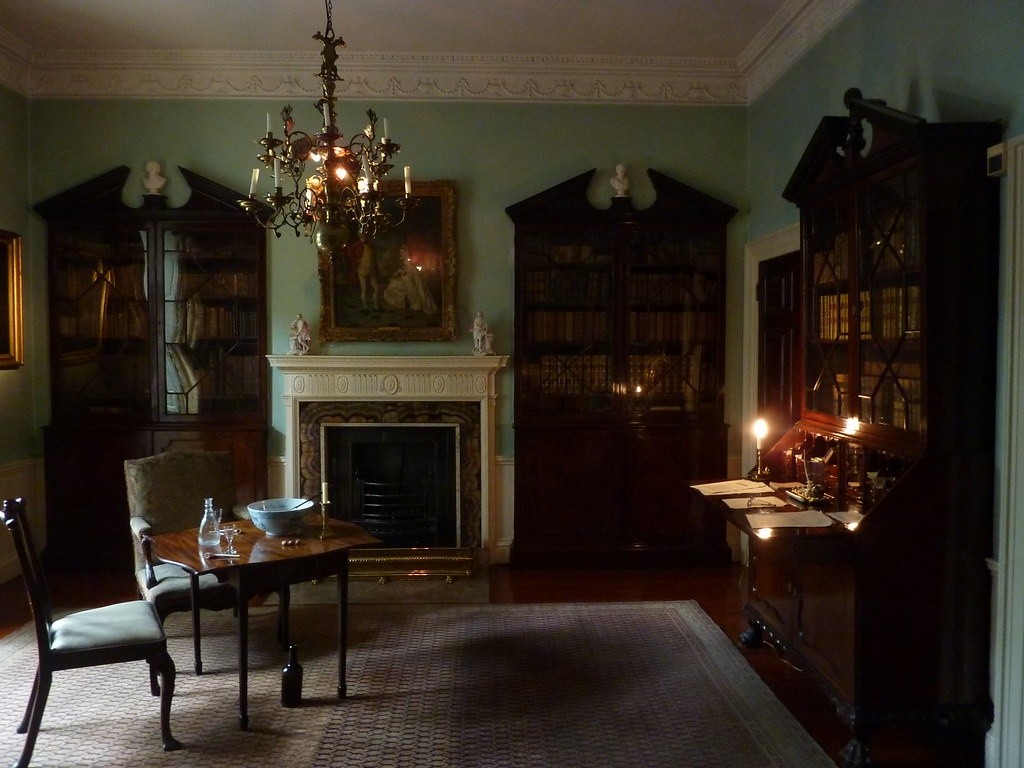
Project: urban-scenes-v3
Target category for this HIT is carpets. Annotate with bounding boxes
[0,597,837,768]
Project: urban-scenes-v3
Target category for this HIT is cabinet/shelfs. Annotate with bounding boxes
[31,161,277,558]
[504,163,739,570]
[680,88,1006,768]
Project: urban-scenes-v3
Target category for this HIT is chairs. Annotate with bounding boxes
[123,449,291,697]
[0,498,184,768]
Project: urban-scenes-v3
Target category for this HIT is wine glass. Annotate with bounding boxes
[213,509,222,533]
[224,526,236,554]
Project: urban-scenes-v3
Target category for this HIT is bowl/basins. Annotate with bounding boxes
[248,498,314,536]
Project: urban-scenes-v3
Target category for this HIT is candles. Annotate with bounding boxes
[748,417,771,450]
[321,482,329,504]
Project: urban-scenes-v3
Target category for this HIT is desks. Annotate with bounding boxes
[152,515,384,732]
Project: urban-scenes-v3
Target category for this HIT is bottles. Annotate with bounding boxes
[198,498,220,546]
[282,644,303,707]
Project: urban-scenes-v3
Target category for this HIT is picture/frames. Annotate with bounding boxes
[0,229,24,366]
[318,180,461,343]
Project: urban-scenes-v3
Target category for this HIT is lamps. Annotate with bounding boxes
[235,0,413,267]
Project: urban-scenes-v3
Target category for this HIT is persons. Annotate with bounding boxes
[611,164,633,196]
[143,160,167,194]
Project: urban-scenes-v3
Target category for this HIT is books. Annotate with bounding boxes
[820,294,848,341]
[166,344,260,398]
[870,212,920,273]
[628,353,722,401]
[163,231,257,340]
[813,233,847,283]
[626,310,719,345]
[859,359,921,432]
[526,309,615,347]
[56,232,148,339]
[524,355,609,408]
[522,242,611,307]
[58,345,151,399]
[833,372,847,417]
[859,287,921,339]
[626,234,718,303]
